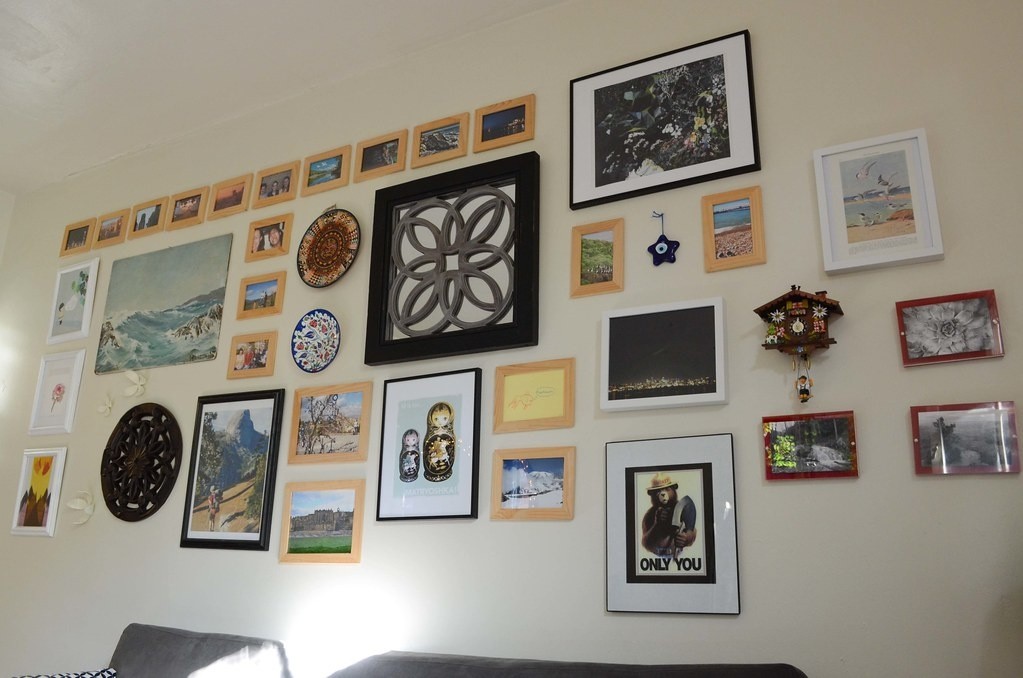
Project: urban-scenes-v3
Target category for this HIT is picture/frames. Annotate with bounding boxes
[9,27,1021,615]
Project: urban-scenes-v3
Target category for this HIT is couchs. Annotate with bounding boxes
[328,651,808,678]
[10,623,289,678]
